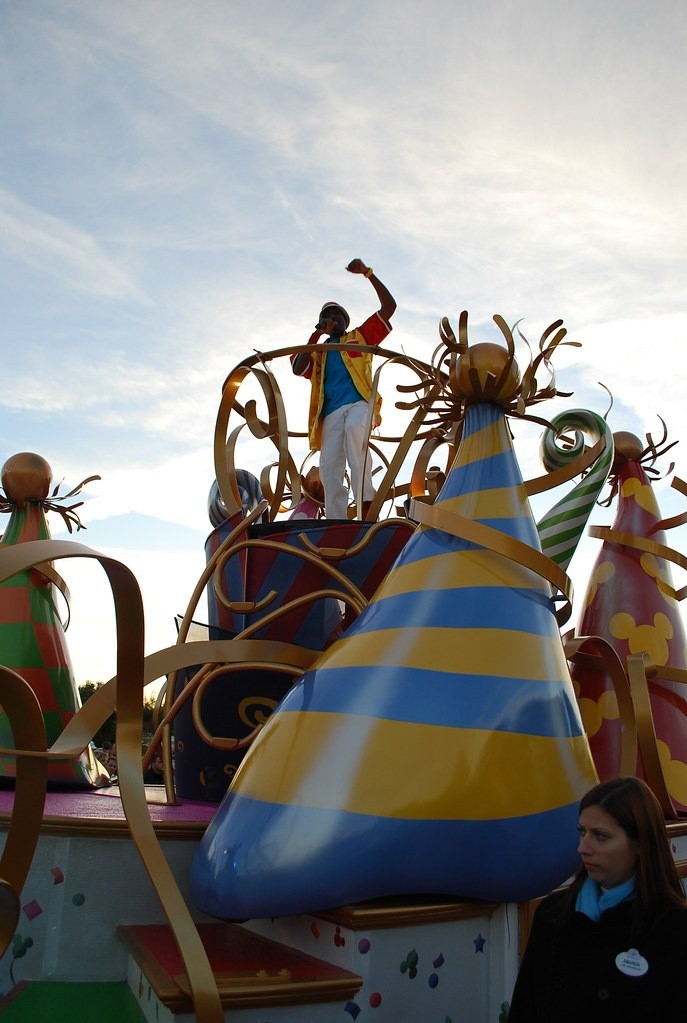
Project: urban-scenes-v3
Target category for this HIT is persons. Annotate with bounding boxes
[90,741,175,785]
[290,259,398,520]
[505,775,687,1023]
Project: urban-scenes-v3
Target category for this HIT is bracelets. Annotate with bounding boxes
[364,268,373,278]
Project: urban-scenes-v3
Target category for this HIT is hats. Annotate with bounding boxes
[319,302,350,333]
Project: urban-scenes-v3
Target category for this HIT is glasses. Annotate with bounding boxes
[321,307,341,319]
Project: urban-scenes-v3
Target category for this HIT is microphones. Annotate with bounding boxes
[315,317,332,329]
